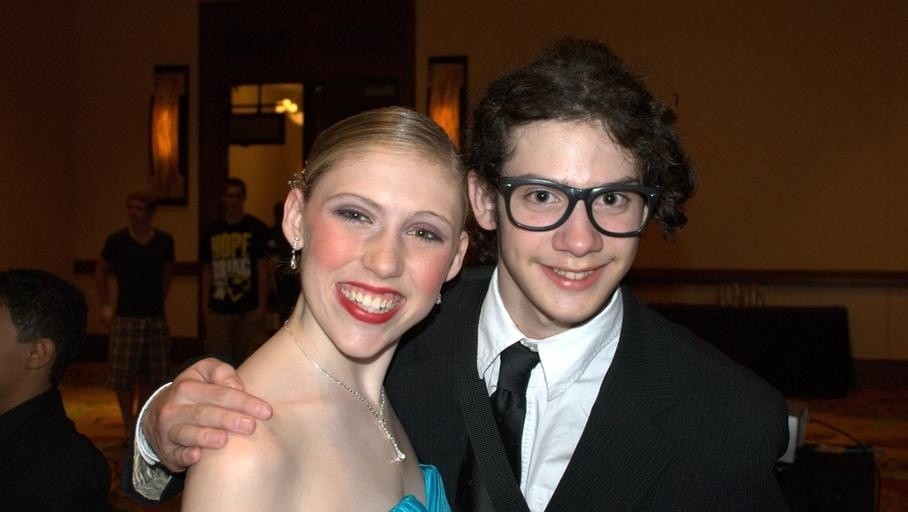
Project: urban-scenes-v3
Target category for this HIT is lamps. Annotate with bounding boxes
[146,62,190,207]
[425,52,468,155]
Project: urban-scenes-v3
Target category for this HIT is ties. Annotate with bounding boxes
[487,341,539,490]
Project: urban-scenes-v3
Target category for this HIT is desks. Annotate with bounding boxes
[645,302,846,400]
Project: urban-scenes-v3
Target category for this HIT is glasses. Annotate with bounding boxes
[484,168,662,238]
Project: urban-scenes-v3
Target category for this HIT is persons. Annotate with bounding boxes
[119,38,791,512]
[0,266,111,512]
[198,177,298,371]
[121,103,471,512]
[96,190,176,449]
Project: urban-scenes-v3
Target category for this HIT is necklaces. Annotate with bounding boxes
[284,317,406,463]
